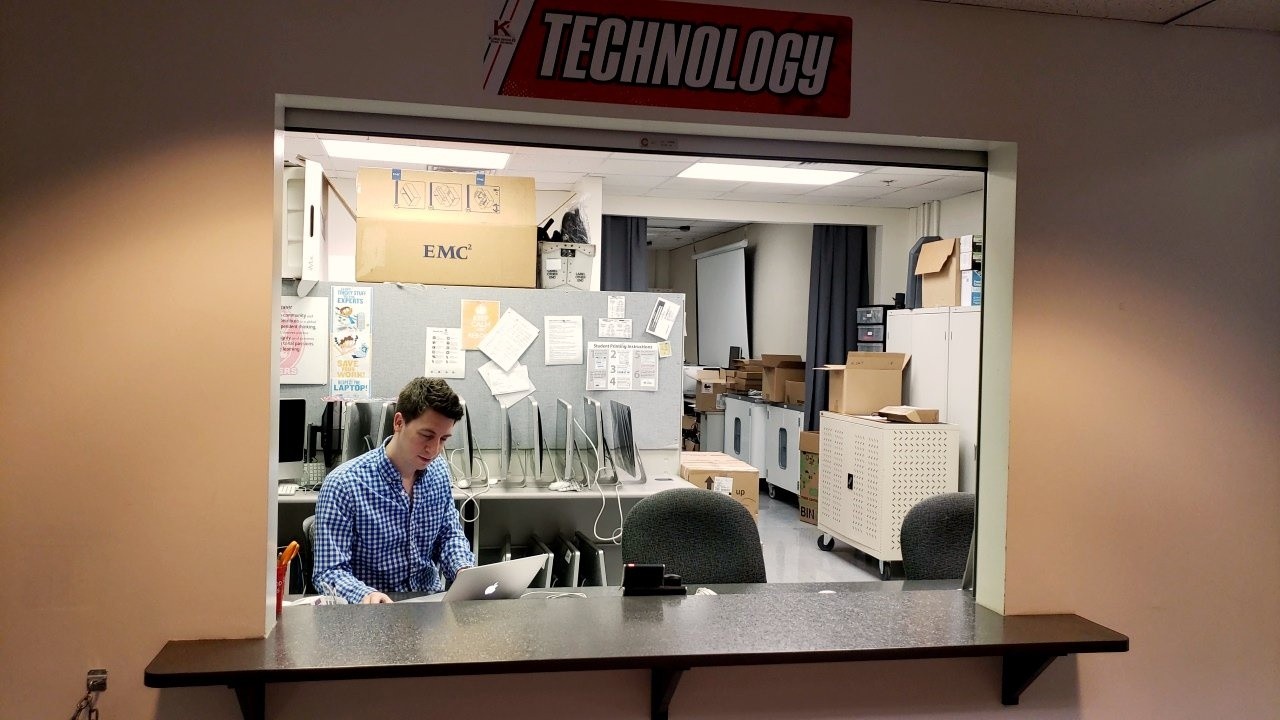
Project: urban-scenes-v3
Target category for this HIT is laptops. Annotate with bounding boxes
[395,552,549,603]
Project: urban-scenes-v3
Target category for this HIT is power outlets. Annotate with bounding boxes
[86,669,107,691]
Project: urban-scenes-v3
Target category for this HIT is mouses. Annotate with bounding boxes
[458,478,471,488]
[549,481,570,490]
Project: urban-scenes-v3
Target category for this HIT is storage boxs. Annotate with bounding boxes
[678,233,982,527]
[354,167,537,289]
[536,241,595,291]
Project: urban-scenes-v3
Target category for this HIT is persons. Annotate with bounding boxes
[310,376,475,604]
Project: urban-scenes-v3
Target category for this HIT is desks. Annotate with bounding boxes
[327,577,962,603]
[280,478,699,586]
[683,397,724,452]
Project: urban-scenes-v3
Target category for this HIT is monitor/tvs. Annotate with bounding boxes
[452,394,646,488]
[498,533,607,589]
[307,400,399,472]
[278,397,306,483]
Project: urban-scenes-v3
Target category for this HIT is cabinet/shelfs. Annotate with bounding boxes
[817,411,958,564]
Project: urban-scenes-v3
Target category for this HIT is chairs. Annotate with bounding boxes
[292,514,317,596]
[900,492,975,580]
[622,487,768,585]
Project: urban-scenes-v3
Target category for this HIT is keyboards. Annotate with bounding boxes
[277,483,298,495]
